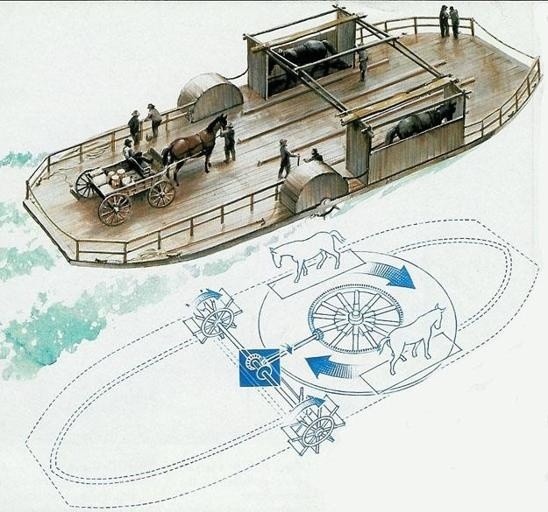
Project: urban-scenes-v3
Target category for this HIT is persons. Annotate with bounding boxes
[302,146,324,163]
[277,138,300,180]
[439,4,450,38]
[121,139,136,159]
[142,103,162,138]
[446,6,460,40]
[127,110,142,148]
[218,121,237,163]
[354,41,369,82]
